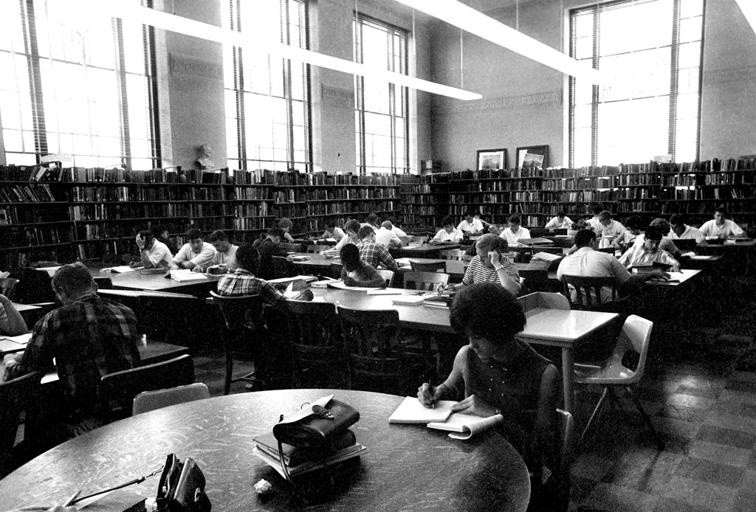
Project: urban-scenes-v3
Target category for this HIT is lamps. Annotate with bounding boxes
[114,0,483,102]
[397,1,610,88]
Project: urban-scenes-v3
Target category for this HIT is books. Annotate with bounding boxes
[389,395,457,424]
[250,424,369,479]
[428,409,505,442]
[99,262,135,274]
[1,154,755,268]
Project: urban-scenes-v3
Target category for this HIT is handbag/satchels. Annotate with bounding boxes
[156,452,212,512]
[273,395,359,459]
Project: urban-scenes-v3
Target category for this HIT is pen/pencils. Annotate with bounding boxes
[429,378,435,410]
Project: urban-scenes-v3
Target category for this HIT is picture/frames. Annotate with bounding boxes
[515,144,549,168]
[475,148,506,171]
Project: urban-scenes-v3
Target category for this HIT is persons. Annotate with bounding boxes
[0,271,28,338]
[7,263,139,438]
[416,283,559,507]
[137,207,755,324]
